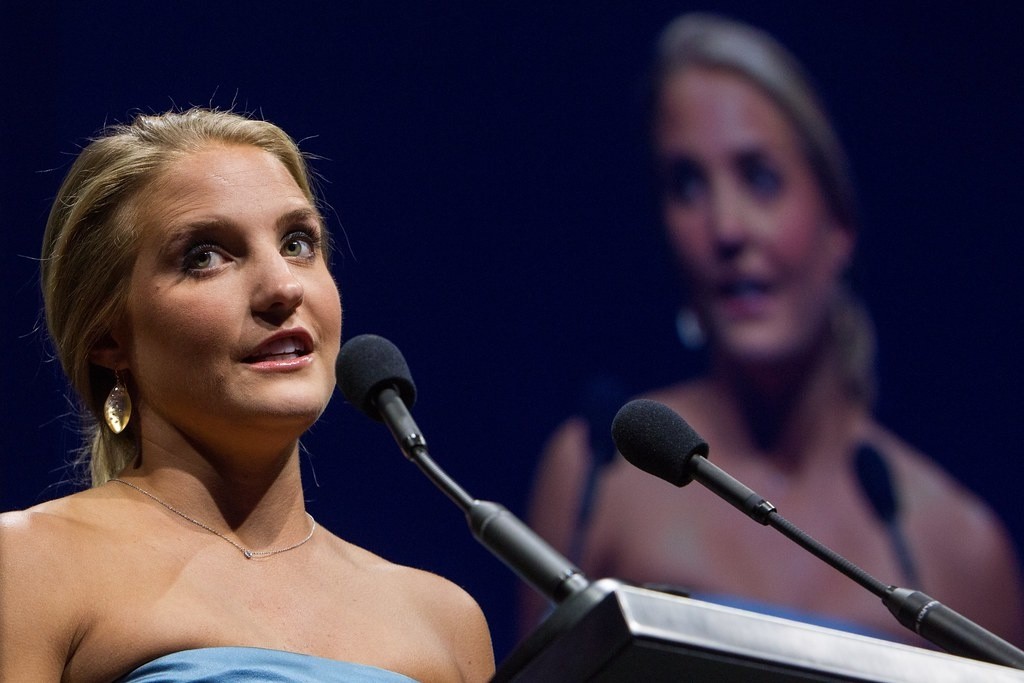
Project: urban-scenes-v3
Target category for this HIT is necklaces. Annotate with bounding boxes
[106,478,315,559]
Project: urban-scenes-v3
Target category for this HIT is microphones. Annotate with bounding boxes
[847,442,923,598]
[610,399,1023,674]
[334,335,589,607]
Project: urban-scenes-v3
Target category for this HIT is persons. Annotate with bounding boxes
[519,14,1021,657]
[0,107,498,683]
